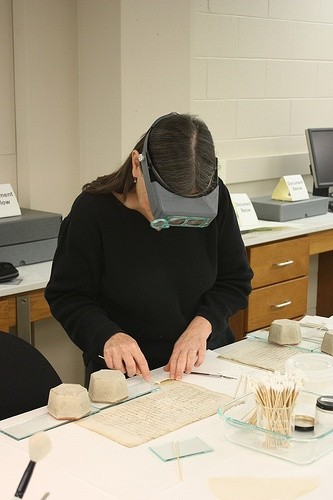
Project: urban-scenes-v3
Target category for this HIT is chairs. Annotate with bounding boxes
[0,330,63,421]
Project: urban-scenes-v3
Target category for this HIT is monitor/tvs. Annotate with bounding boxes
[304,127,333,188]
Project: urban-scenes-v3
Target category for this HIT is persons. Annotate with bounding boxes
[44,112,254,380]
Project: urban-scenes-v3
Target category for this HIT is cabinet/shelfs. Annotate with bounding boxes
[228,237,309,341]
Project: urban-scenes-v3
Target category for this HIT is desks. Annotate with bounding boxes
[0,315,333,500]
[0,211,333,344]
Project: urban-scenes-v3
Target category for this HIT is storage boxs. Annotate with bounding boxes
[0,208,62,266]
[218,389,333,464]
[250,194,329,221]
